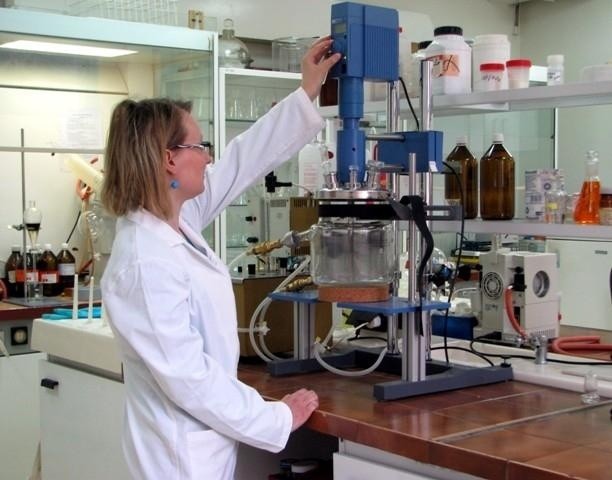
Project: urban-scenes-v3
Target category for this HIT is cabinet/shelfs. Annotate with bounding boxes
[307,76,612,401]
[164,33,328,273]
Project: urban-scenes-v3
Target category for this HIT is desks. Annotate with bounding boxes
[238,322,611,480]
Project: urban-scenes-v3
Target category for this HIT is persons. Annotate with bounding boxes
[102,38,341,480]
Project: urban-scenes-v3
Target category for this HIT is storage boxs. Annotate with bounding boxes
[34,353,132,480]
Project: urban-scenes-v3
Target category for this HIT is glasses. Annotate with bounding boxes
[168,141,212,158]
